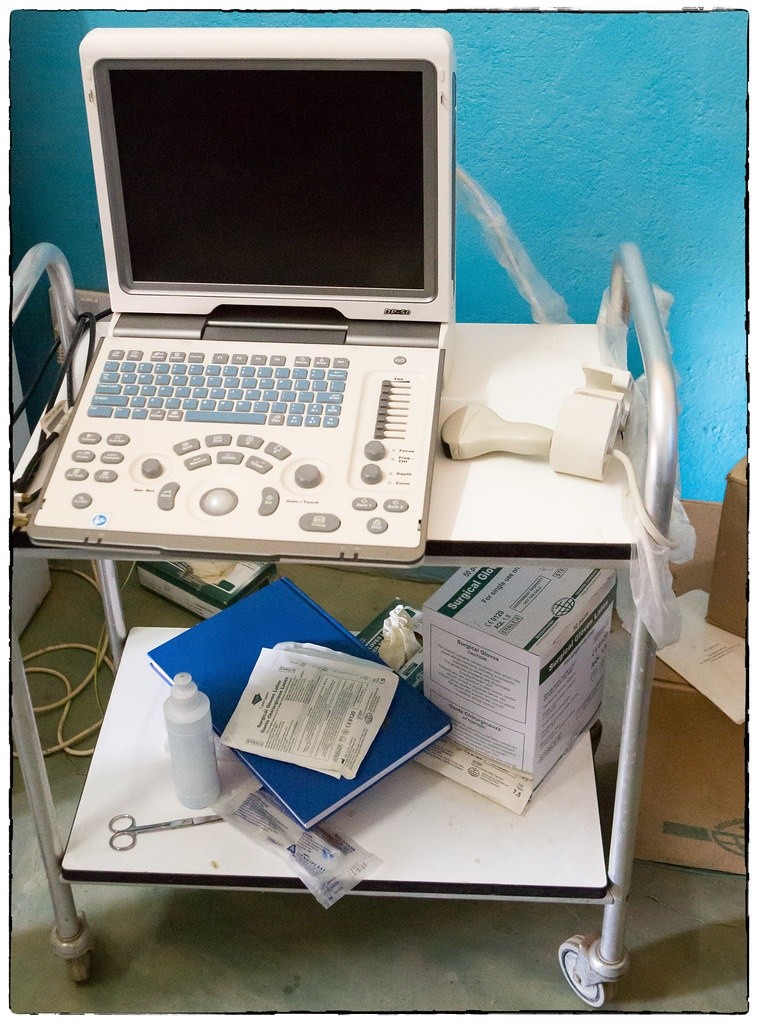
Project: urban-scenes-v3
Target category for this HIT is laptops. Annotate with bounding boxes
[27,29,456,570]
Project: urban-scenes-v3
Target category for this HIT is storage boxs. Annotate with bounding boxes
[704,458,748,638]
[355,592,595,815]
[634,500,746,877]
[137,561,277,620]
[420,564,617,776]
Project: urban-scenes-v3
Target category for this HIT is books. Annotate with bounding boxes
[148,575,454,832]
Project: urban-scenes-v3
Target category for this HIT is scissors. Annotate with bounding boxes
[109,814,224,852]
